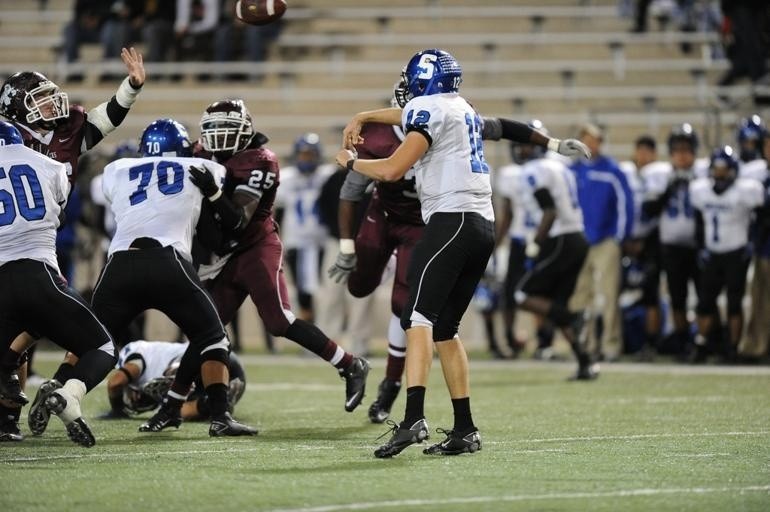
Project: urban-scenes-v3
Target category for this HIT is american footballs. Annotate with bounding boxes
[233,0,288,26]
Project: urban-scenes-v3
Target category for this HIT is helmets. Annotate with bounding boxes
[668,123,698,152]
[400,48,462,100]
[1,69,70,132]
[197,97,257,154]
[707,145,739,187]
[122,386,157,412]
[1,122,23,145]
[391,76,414,109]
[737,114,765,153]
[138,119,194,156]
[291,134,324,175]
[511,117,550,164]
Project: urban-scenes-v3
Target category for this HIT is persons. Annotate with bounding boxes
[336,49,496,458]
[48,1,285,83]
[326,118,591,423]
[474,120,770,380]
[27,119,259,436]
[101,339,246,418]
[0,47,146,435]
[192,100,371,412]
[268,133,374,360]
[0,121,119,447]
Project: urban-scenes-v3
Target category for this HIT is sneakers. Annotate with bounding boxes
[573,311,593,350]
[0,406,27,444]
[207,411,260,437]
[570,363,605,381]
[142,375,198,399]
[28,379,65,438]
[425,426,482,456]
[368,382,399,424]
[198,378,243,420]
[337,357,370,412]
[139,403,184,433]
[373,415,432,458]
[0,361,30,406]
[45,386,96,447]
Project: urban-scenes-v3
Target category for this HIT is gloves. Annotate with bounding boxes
[189,165,223,202]
[328,239,359,285]
[551,136,593,160]
[111,401,138,419]
